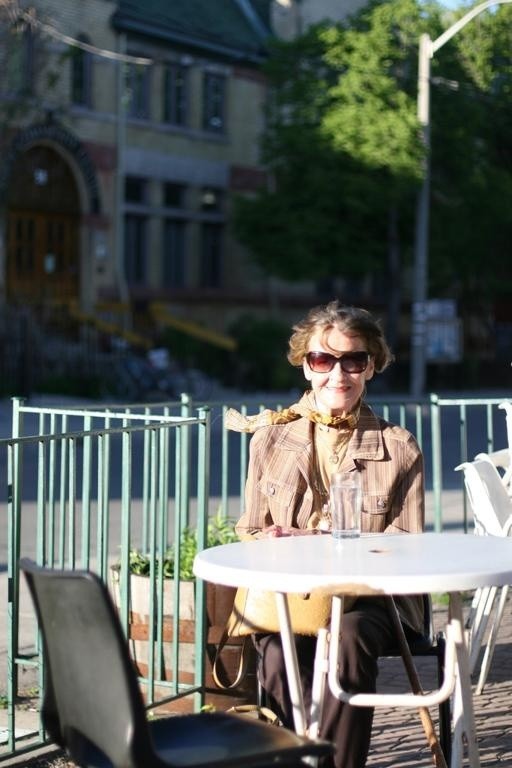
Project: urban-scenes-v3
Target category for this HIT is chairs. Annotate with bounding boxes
[19,557,336,768]
[257,592,452,768]
[454,401,512,696]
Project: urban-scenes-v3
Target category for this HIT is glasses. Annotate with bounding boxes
[303,351,373,374]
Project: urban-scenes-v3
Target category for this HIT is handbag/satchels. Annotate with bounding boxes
[226,533,332,638]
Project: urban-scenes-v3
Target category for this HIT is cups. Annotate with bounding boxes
[329,470,362,538]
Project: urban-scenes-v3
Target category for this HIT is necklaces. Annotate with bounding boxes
[311,413,351,464]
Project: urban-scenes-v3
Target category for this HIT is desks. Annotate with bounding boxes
[194,530,512,768]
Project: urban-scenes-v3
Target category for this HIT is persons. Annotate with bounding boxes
[229,295,426,768]
